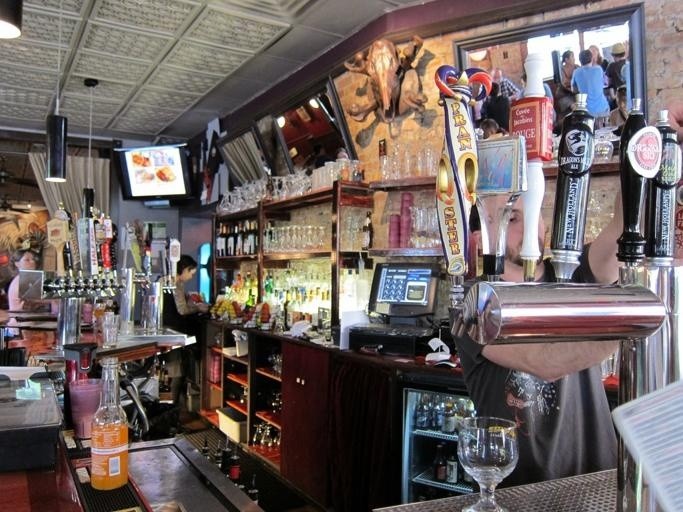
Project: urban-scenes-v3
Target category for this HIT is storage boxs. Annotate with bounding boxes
[216,407,247,444]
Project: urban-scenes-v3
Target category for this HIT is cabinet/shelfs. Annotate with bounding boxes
[368,177,445,257]
[202,180,371,511]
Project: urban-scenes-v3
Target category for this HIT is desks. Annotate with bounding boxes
[95,326,197,441]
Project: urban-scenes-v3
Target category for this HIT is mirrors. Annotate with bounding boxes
[269,73,359,176]
[215,125,277,186]
[451,0,647,178]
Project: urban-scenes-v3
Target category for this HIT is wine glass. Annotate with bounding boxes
[253,353,282,450]
[218,158,362,215]
[261,215,362,253]
[456,415,520,512]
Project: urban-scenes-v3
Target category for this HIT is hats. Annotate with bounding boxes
[611,43,626,54]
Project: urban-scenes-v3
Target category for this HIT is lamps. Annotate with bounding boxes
[45,0,68,183]
[83,78,99,217]
[0,0,23,39]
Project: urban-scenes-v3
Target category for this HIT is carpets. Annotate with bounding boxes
[184,429,309,512]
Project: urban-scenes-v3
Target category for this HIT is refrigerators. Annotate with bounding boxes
[394,369,482,505]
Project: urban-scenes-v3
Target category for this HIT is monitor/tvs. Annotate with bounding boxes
[369,263,441,318]
[112,143,193,201]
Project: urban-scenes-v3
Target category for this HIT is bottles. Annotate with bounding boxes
[416,393,473,485]
[215,220,258,259]
[361,211,375,250]
[90,357,128,491]
[202,431,259,504]
[213,269,330,342]
[146,357,171,391]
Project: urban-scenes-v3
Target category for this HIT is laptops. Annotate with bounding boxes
[19,269,47,300]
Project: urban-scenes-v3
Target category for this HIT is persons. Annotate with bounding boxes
[7,249,36,312]
[163,255,209,405]
[479,118,500,138]
[493,69,521,105]
[481,83,510,134]
[556,40,629,127]
[452,100,683,493]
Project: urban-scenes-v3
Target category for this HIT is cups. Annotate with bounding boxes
[67,378,100,441]
[378,141,442,184]
[385,189,441,250]
[96,310,133,349]
